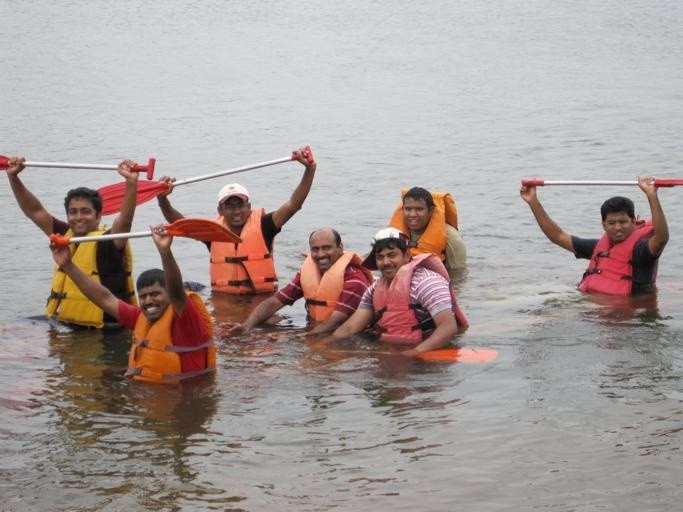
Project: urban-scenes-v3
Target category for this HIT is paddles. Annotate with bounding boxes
[50,218,243,247]
[96,146,313,215]
[521,179,683,188]
[332,350,497,363]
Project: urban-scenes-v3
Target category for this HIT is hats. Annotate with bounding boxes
[361,228,416,271]
[217,184,249,204]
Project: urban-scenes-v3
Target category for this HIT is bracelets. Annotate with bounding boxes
[158,201,170,208]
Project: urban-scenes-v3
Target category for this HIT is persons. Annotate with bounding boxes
[518,175,670,300]
[385,186,468,272]
[219,228,378,345]
[300,228,470,357]
[47,223,218,388]
[156,146,317,300]
[4,154,140,336]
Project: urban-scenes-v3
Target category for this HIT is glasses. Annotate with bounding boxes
[220,202,249,208]
